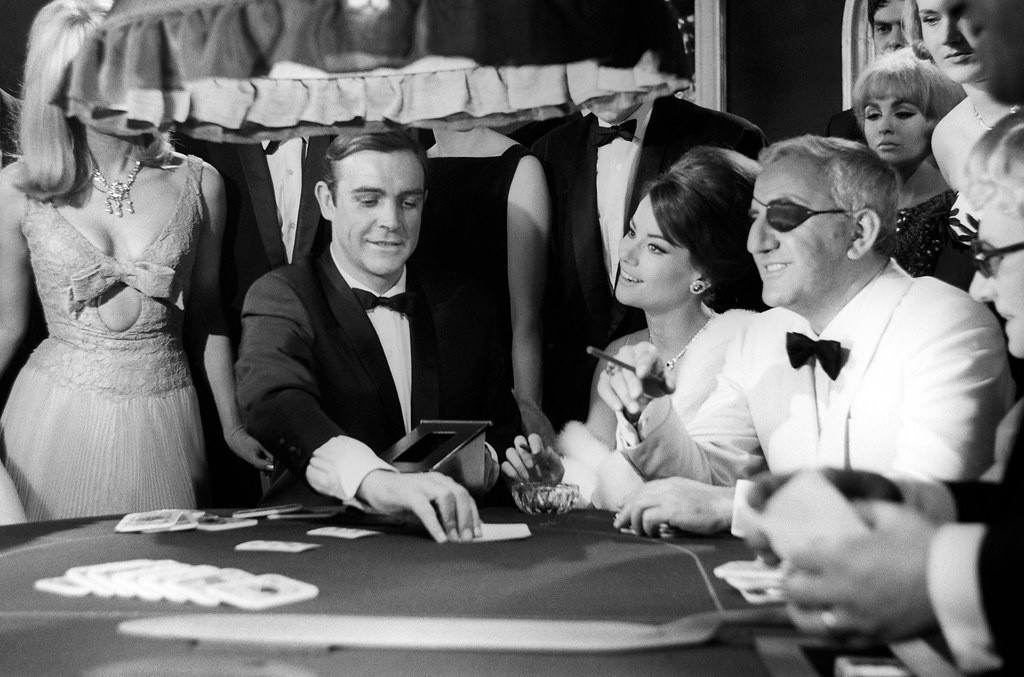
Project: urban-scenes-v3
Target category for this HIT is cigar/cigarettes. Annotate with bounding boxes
[588,348,663,382]
[519,444,535,456]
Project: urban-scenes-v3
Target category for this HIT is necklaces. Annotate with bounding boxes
[648,307,715,373]
[91,159,146,216]
[971,102,995,131]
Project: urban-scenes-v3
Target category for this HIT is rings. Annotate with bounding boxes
[604,362,622,376]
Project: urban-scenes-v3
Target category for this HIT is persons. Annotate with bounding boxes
[779,0,1024,288]
[597,136,1009,558]
[0,1,278,525]
[498,148,762,510]
[237,127,501,545]
[222,89,769,504]
[738,102,1024,677]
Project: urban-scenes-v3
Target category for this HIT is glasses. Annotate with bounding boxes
[971,240,1024,280]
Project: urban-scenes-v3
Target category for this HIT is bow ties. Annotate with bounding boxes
[594,119,637,147]
[350,289,410,315]
[786,333,847,380]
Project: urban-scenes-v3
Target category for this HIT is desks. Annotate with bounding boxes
[0,494,893,677]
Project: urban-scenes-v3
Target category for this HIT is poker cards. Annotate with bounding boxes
[714,558,786,604]
[235,539,322,553]
[757,468,869,557]
[306,526,379,539]
[458,524,531,543]
[34,557,319,613]
[113,508,259,531]
